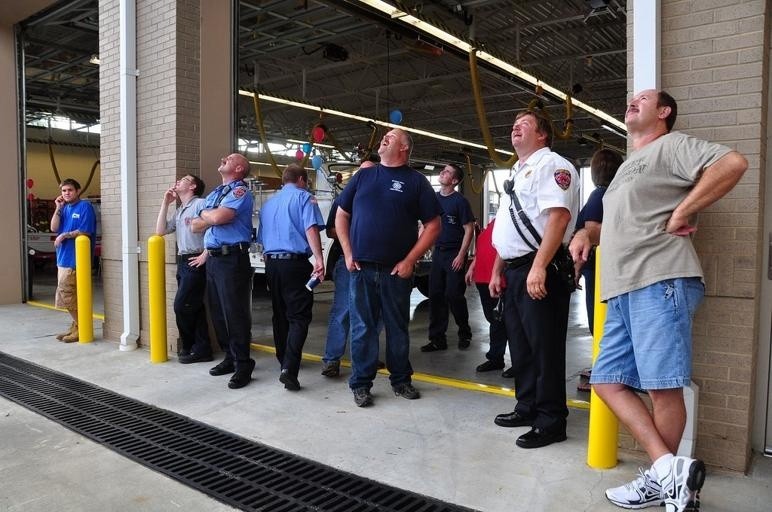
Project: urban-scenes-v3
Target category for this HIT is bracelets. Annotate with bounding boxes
[53,208,61,216]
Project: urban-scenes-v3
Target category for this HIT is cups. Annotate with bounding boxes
[304,271,322,291]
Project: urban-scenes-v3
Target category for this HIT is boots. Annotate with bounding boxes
[56,320,79,342]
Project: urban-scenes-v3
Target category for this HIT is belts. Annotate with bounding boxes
[207,250,222,256]
[504,254,535,269]
[268,254,298,259]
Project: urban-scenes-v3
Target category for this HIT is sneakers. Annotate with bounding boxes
[606,469,665,509]
[477,360,505,371]
[662,456,706,512]
[458,335,471,349]
[321,360,420,408]
[421,341,447,352]
[502,366,514,378]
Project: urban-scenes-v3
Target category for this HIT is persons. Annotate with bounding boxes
[571,147,625,392]
[321,153,385,378]
[334,129,445,407]
[488,111,580,448]
[569,88,748,512]
[465,220,517,377]
[49,179,97,344]
[418,164,475,351]
[191,153,255,389]
[155,175,215,363]
[256,164,327,389]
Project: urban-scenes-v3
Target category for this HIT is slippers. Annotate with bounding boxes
[577,368,592,392]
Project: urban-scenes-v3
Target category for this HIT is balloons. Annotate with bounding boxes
[312,156,323,171]
[296,149,304,161]
[390,109,402,124]
[313,127,324,144]
[302,143,313,154]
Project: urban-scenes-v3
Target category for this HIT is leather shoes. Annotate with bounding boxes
[516,426,566,448]
[179,345,256,388]
[279,369,300,391]
[495,411,534,426]
